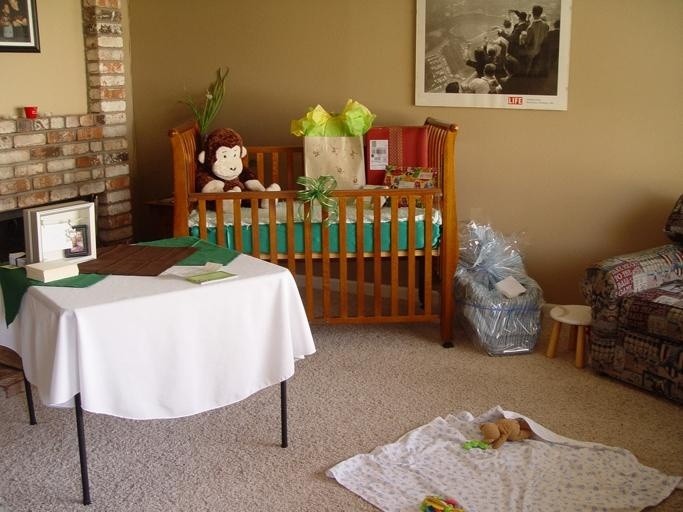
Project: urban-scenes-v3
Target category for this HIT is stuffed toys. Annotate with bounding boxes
[196,127,281,214]
[481,418,533,448]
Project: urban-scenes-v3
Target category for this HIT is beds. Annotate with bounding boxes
[165,114,460,350]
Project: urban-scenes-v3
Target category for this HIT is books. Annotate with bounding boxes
[185,271,238,285]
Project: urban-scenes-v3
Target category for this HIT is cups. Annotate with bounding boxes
[24,107,38,118]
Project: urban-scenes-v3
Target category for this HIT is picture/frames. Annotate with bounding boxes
[65,223,89,259]
[0,0,42,54]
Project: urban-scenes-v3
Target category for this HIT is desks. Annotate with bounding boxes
[1,238,308,507]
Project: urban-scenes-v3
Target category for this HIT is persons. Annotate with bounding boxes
[0,0,29,39]
[445,5,559,94]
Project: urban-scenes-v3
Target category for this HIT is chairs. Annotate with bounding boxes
[579,194,683,409]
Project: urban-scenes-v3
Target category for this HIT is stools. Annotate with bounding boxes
[547,301,594,370]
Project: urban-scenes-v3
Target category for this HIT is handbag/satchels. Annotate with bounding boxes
[303,136,366,191]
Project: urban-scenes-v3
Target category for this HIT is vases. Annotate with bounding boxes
[24,106,38,119]
